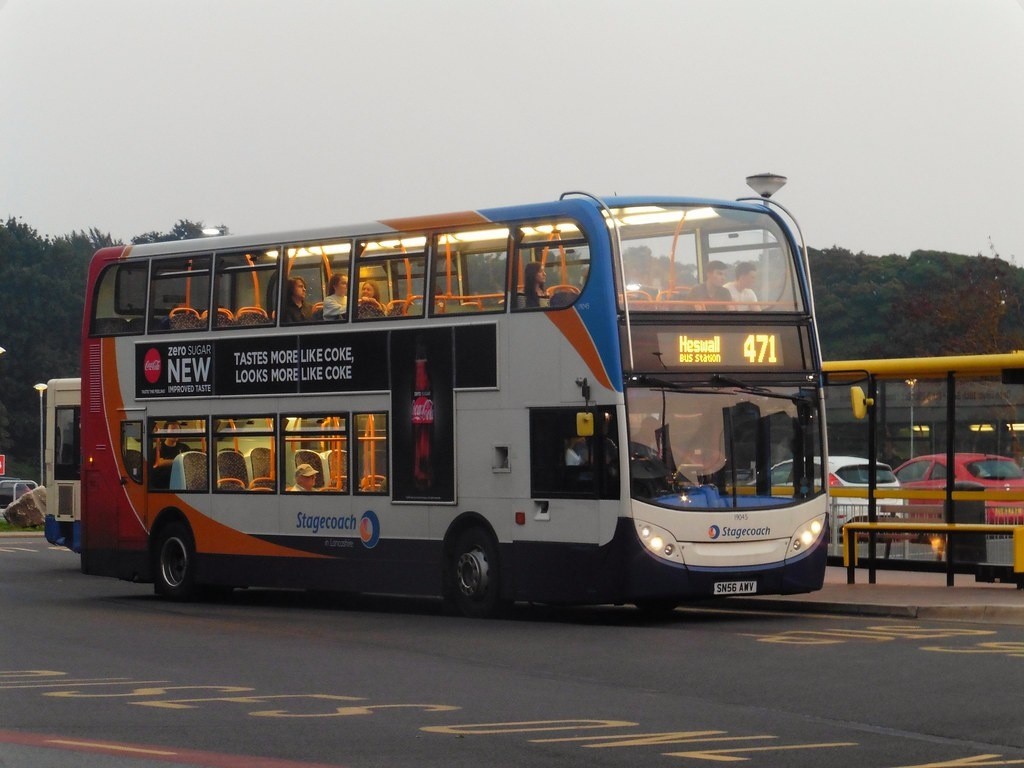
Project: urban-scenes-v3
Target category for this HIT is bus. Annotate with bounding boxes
[80,192,874,620]
[44,380,81,552]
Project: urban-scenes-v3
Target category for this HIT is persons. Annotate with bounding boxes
[687,261,734,312]
[517,262,550,308]
[291,464,319,491]
[361,281,387,312]
[631,416,694,471]
[152,421,191,489]
[323,273,348,321]
[281,275,317,323]
[722,262,762,312]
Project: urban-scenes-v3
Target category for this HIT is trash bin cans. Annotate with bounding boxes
[941,481,987,563]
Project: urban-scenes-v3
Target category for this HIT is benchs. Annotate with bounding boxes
[843,521,1024,590]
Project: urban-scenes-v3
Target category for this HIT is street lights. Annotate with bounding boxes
[745,174,788,309]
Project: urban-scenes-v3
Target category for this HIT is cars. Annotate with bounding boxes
[746,456,904,541]
[893,453,1024,535]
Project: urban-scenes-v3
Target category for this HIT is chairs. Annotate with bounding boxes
[92,285,693,335]
[565,435,619,494]
[124,447,387,495]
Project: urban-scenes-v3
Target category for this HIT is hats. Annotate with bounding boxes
[295,464,320,477]
